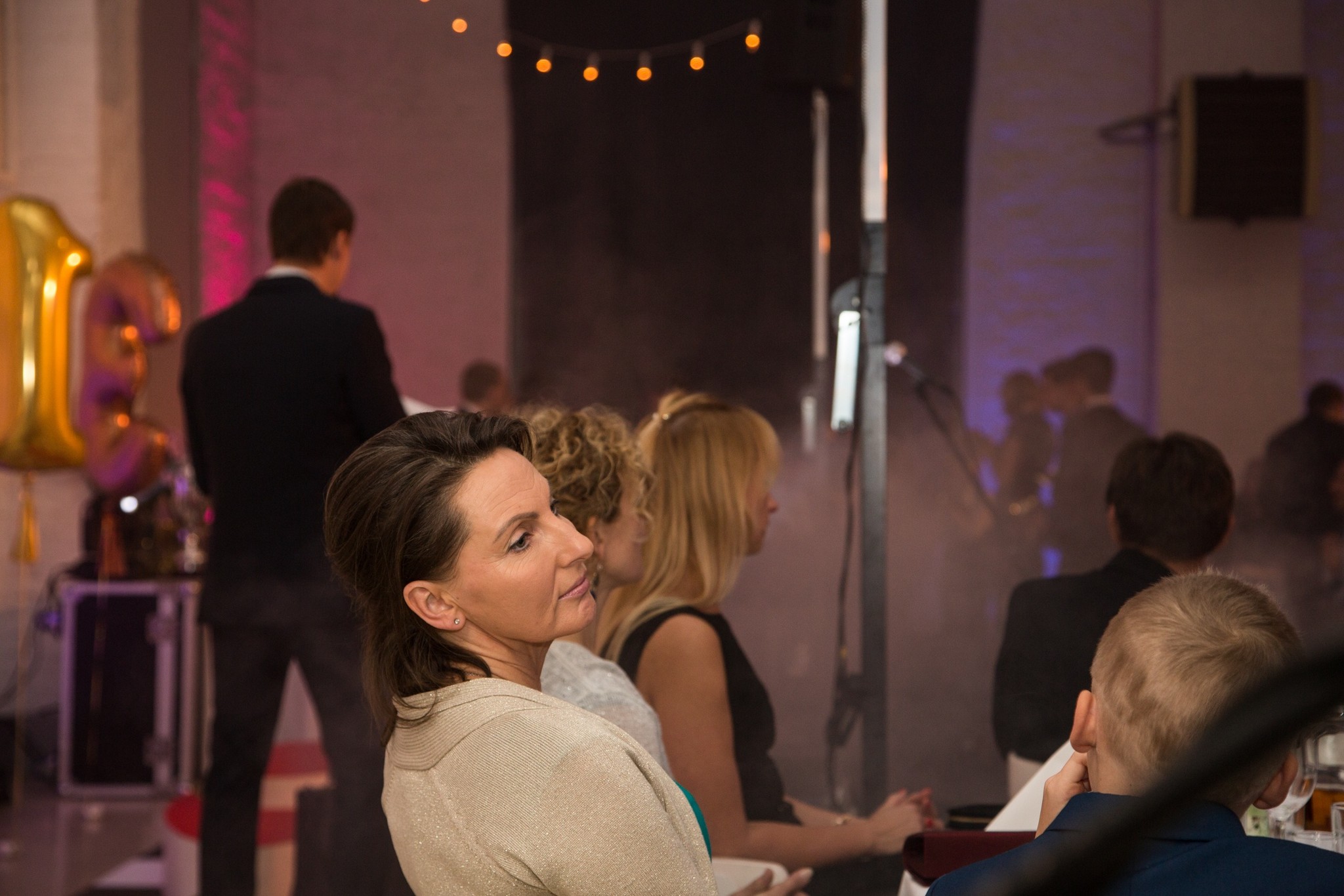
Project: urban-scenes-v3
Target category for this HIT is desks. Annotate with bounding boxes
[56,574,219,801]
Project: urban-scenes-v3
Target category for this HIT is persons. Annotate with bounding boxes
[319,406,814,896]
[989,428,1236,765]
[924,572,1344,896]
[178,176,410,896]
[990,344,1341,643]
[572,390,947,896]
[444,359,501,421]
[505,402,796,895]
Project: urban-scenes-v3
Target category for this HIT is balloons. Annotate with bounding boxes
[0,190,94,470]
[78,249,187,491]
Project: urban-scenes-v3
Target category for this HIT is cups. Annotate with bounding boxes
[1331,801,1344,855]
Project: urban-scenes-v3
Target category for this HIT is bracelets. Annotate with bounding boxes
[832,815,856,828]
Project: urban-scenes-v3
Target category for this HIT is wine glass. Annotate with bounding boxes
[1268,764,1318,838]
